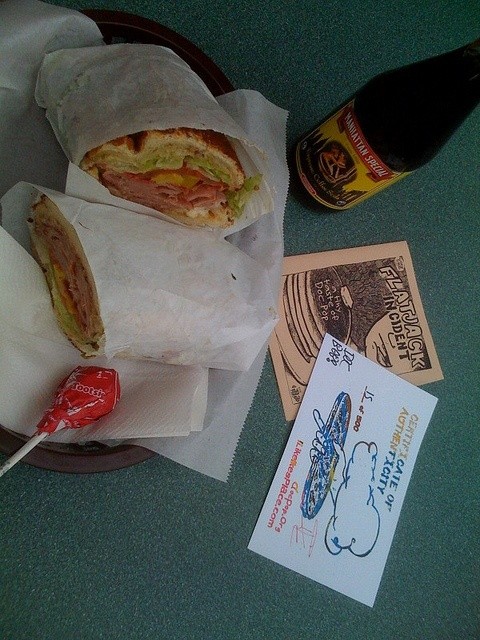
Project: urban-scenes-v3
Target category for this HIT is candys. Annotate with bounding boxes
[40,360,121,434]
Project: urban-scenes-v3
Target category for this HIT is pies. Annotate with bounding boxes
[35,44,256,229]
[26,191,279,357]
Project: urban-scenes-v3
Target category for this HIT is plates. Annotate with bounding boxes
[2,7,249,474]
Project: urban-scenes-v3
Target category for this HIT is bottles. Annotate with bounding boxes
[288,41,479,212]
